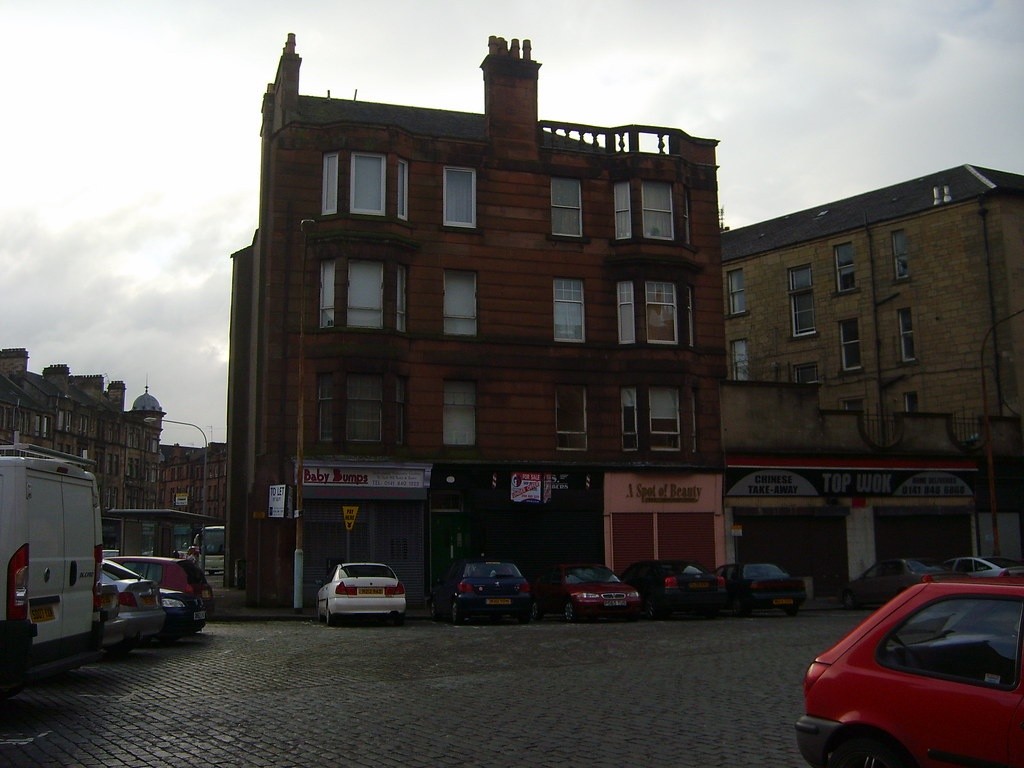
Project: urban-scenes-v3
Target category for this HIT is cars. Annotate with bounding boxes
[713,561,806,617]
[620,559,729,621]
[105,556,215,615]
[531,560,642,623]
[101,560,207,636]
[430,562,534,626]
[839,558,972,609]
[942,556,1024,577]
[316,561,406,628]
[794,570,1024,768]
[100,570,166,655]
[100,582,126,648]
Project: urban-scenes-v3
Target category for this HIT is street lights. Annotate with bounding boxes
[144,418,207,574]
[293,218,316,613]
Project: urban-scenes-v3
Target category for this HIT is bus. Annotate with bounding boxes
[194,526,225,574]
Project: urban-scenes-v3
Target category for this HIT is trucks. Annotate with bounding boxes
[0,442,108,696]
